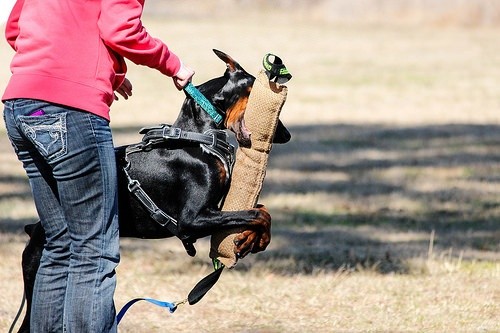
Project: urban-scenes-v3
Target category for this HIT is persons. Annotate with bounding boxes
[1,0,195,333]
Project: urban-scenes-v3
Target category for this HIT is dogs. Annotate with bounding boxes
[18,49,291,333]
